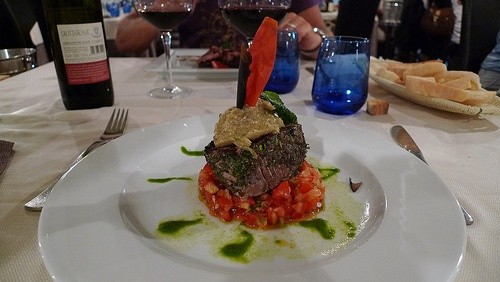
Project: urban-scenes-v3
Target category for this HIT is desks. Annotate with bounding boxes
[0,57,500,282]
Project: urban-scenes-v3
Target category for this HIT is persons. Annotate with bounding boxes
[115,0,334,61]
[335,0,500,91]
[0,0,53,77]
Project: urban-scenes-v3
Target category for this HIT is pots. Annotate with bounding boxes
[0,48,36,76]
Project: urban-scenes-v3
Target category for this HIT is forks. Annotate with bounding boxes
[24,108,129,210]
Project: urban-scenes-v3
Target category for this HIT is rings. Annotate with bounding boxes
[288,23,296,28]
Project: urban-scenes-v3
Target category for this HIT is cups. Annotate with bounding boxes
[311,36,370,116]
[262,28,300,94]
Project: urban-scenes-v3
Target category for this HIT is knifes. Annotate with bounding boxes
[391,124,474,226]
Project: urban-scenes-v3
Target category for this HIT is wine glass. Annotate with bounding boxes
[219,0,293,52]
[132,0,198,100]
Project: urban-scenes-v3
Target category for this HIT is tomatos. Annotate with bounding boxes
[196,160,326,226]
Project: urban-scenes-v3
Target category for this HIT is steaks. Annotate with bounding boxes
[197,45,241,69]
[203,120,307,198]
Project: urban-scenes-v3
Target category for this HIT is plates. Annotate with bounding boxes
[145,48,240,79]
[35,111,467,282]
[371,71,500,114]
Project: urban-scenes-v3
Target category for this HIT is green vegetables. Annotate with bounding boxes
[259,90,299,123]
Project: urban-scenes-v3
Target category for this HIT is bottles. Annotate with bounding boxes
[34,0,116,111]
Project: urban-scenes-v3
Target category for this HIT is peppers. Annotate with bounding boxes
[246,15,278,106]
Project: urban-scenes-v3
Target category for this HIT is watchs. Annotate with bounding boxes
[300,27,329,56]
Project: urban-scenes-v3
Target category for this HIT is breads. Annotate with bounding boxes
[366,99,390,115]
[374,61,497,108]
